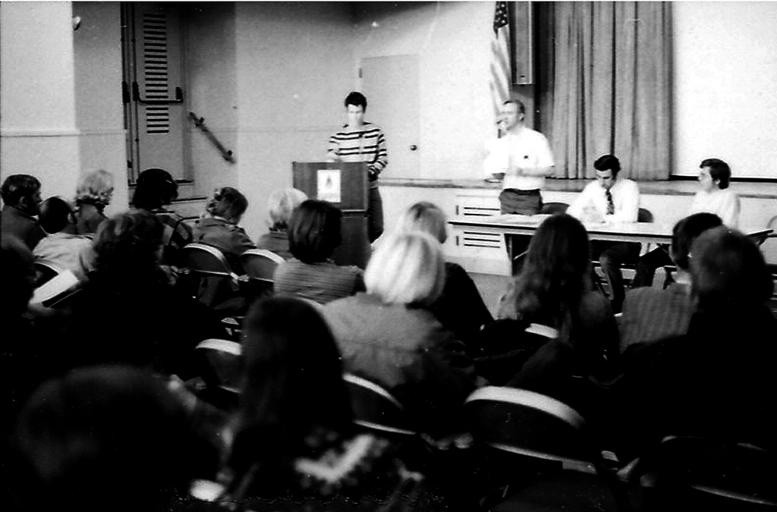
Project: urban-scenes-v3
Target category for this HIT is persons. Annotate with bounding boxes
[0,153,777,511]
[327,90,388,245]
[494,99,556,279]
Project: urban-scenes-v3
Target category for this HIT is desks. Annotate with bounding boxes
[446,213,674,248]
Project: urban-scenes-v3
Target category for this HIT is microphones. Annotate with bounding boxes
[358,133,365,162]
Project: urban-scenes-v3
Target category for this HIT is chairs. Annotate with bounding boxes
[32,258,61,281]
[180,242,285,318]
[341,372,422,439]
[194,336,245,410]
[465,384,776,512]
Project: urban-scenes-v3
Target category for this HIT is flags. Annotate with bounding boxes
[489,1,513,118]
